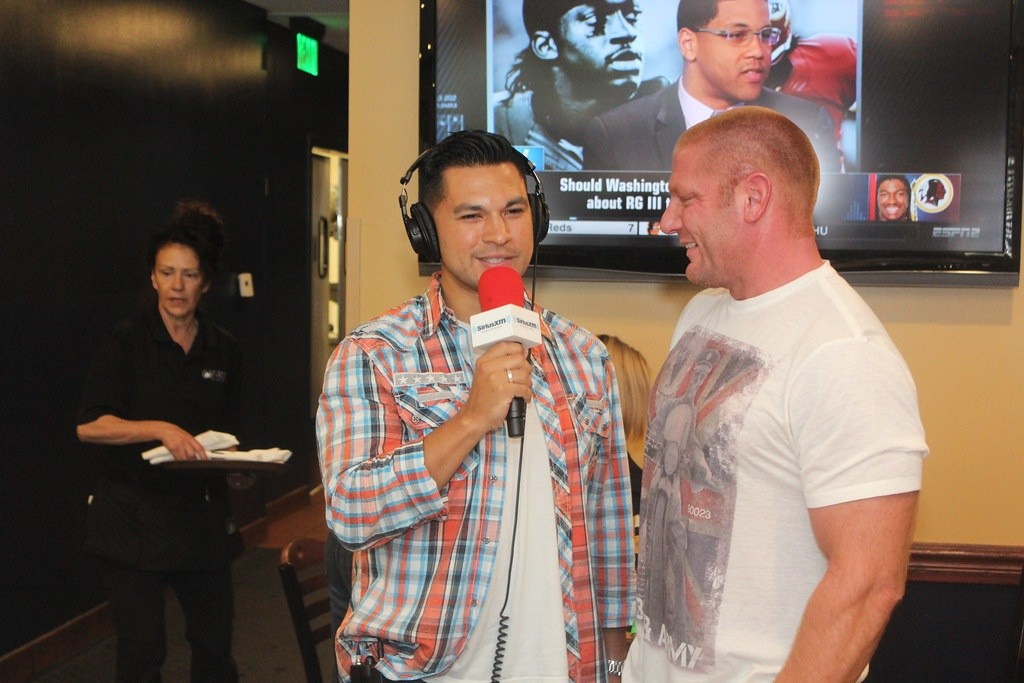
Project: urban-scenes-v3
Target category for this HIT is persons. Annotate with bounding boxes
[316,129,639,683]
[70,203,240,683]
[595,334,652,571]
[491,0,646,171]
[582,0,840,172]
[621,106,929,683]
[763,0,857,144]
[876,175,911,222]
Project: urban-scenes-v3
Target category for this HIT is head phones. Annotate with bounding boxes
[398,126,550,262]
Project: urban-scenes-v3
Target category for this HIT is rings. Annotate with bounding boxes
[507,370,513,383]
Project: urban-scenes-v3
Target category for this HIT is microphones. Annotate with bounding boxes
[468,266,544,439]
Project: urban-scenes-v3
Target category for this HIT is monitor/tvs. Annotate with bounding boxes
[418,0,1023,285]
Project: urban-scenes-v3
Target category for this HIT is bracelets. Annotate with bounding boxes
[606,660,622,676]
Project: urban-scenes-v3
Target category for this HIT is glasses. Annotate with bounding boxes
[690,24,782,47]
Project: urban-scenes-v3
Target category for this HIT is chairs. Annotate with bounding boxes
[278,537,330,683]
